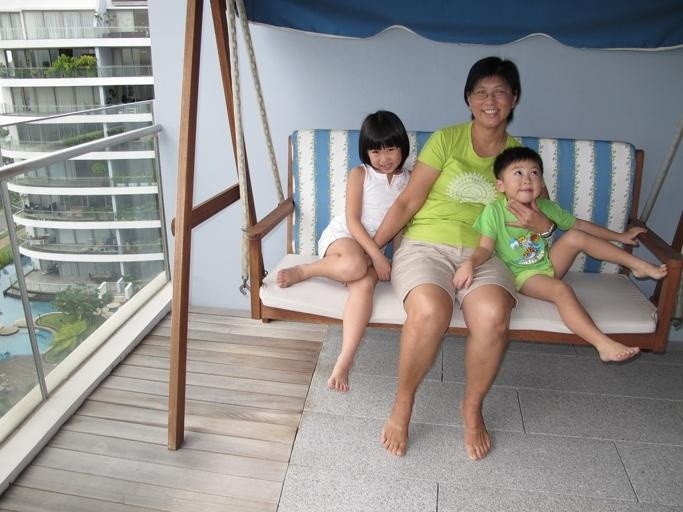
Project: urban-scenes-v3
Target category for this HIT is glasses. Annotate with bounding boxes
[472,89,507,98]
